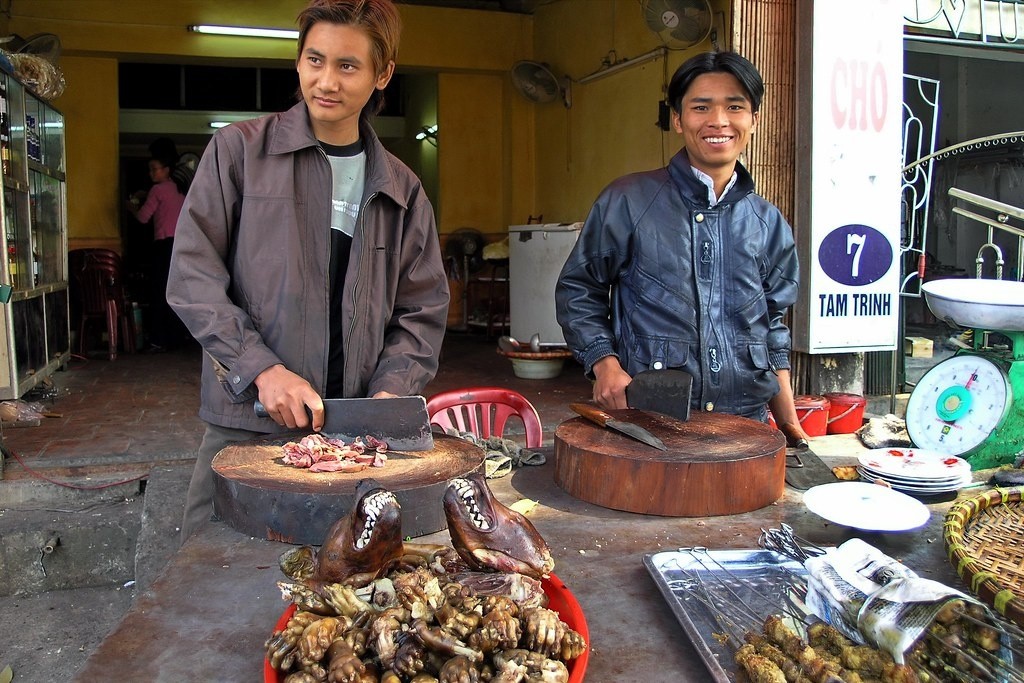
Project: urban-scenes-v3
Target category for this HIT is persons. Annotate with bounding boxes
[554,50,811,447]
[165,0,451,549]
[136,149,194,349]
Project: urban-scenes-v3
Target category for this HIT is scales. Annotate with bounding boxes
[905,278,1024,472]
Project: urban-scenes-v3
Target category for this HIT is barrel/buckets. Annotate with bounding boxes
[829,392,868,435]
[793,394,829,436]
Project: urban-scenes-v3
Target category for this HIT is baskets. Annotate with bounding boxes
[942,486,1024,628]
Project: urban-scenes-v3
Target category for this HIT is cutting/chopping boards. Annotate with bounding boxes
[210,426,486,545]
[552,408,787,516]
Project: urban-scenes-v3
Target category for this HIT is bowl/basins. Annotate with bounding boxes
[919,279,1024,332]
[508,358,567,381]
[263,573,589,682]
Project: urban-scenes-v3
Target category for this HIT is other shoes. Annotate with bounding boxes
[150,340,168,353]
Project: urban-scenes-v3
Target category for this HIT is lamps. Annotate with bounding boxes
[187,24,301,41]
[579,47,665,85]
[209,121,233,128]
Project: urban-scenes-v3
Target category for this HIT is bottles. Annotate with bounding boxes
[0,111,42,292]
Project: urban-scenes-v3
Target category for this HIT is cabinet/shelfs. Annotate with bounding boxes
[0,64,69,399]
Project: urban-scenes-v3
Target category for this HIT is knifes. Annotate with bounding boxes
[626,368,693,423]
[568,402,668,452]
[254,395,434,451]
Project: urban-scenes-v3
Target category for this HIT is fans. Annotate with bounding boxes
[510,60,572,108]
[640,0,719,51]
[445,227,488,336]
[415,125,440,147]
[5,30,59,61]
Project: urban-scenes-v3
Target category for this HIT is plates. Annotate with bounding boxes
[801,446,973,538]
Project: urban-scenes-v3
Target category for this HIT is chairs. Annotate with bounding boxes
[67,247,137,362]
[425,388,545,452]
